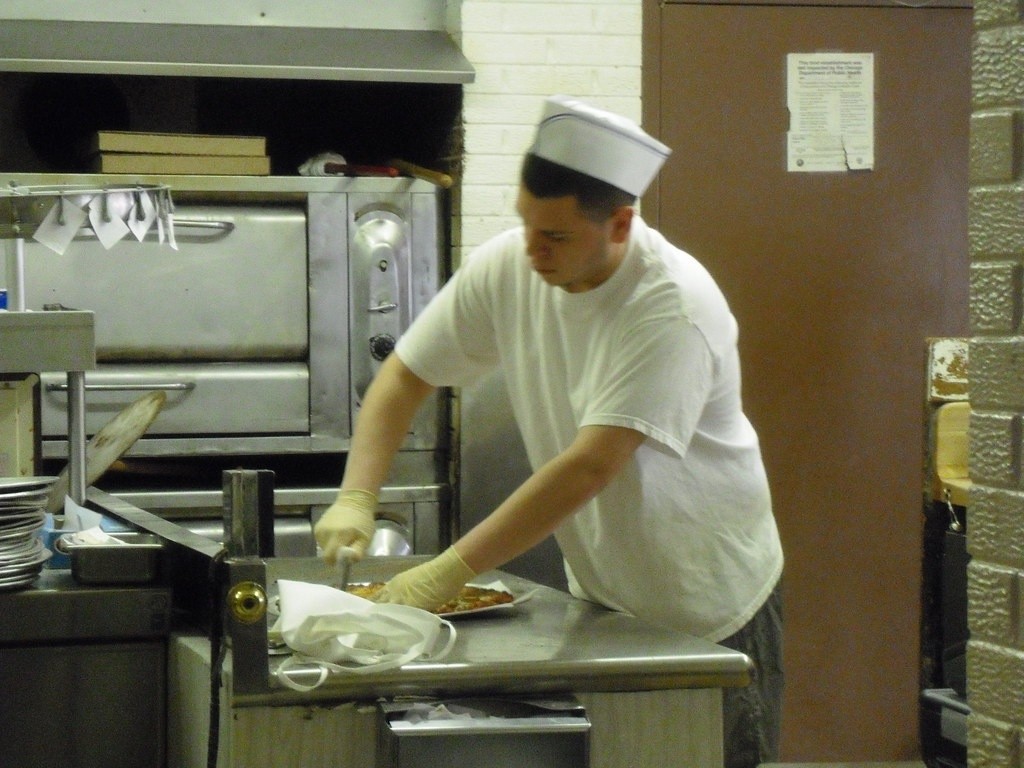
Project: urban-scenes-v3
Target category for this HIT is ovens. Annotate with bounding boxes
[0,169,445,485]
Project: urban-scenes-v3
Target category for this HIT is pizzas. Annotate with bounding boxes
[345,580,515,614]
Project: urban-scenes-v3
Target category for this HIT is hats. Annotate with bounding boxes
[530,93,674,198]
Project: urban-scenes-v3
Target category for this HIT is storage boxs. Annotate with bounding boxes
[90,129,271,176]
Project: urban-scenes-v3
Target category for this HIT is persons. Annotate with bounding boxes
[312,97,795,768]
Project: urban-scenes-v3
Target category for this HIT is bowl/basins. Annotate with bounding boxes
[61,531,167,585]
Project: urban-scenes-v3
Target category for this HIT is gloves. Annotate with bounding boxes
[311,488,379,566]
[368,545,476,611]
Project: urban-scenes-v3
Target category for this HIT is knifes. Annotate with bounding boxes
[335,545,350,590]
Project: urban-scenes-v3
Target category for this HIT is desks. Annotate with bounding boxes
[168,554,753,768]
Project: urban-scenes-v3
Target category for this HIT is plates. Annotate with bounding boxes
[0,475,61,587]
